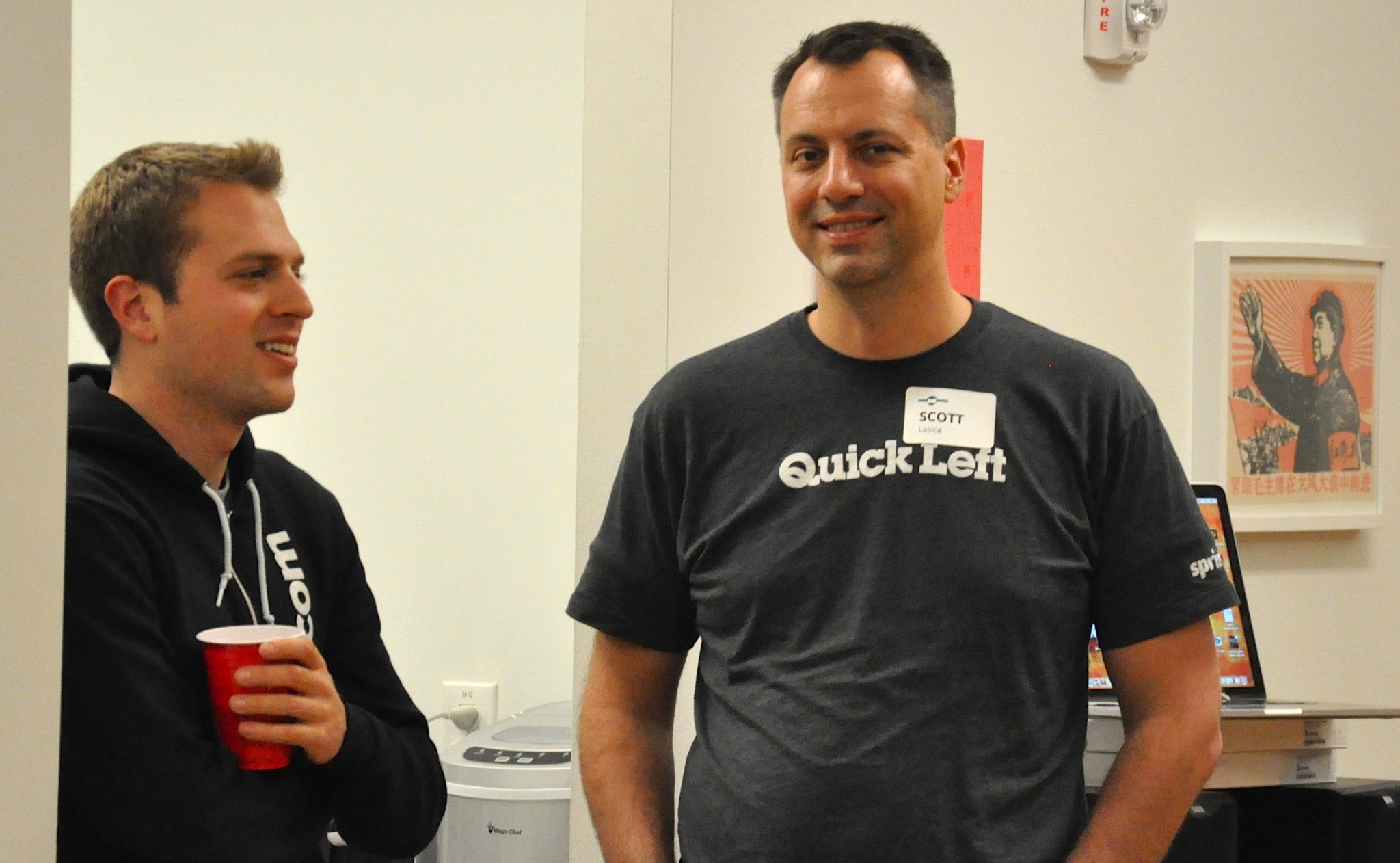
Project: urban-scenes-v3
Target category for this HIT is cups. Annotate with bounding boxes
[192,622,303,770]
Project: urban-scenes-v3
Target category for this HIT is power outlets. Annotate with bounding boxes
[441,682,499,745]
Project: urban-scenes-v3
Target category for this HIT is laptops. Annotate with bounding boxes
[1088,485,1400,716]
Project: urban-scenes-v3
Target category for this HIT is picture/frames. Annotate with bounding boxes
[1192,238,1390,534]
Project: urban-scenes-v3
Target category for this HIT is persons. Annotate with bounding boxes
[56,137,446,863]
[561,14,1245,863]
